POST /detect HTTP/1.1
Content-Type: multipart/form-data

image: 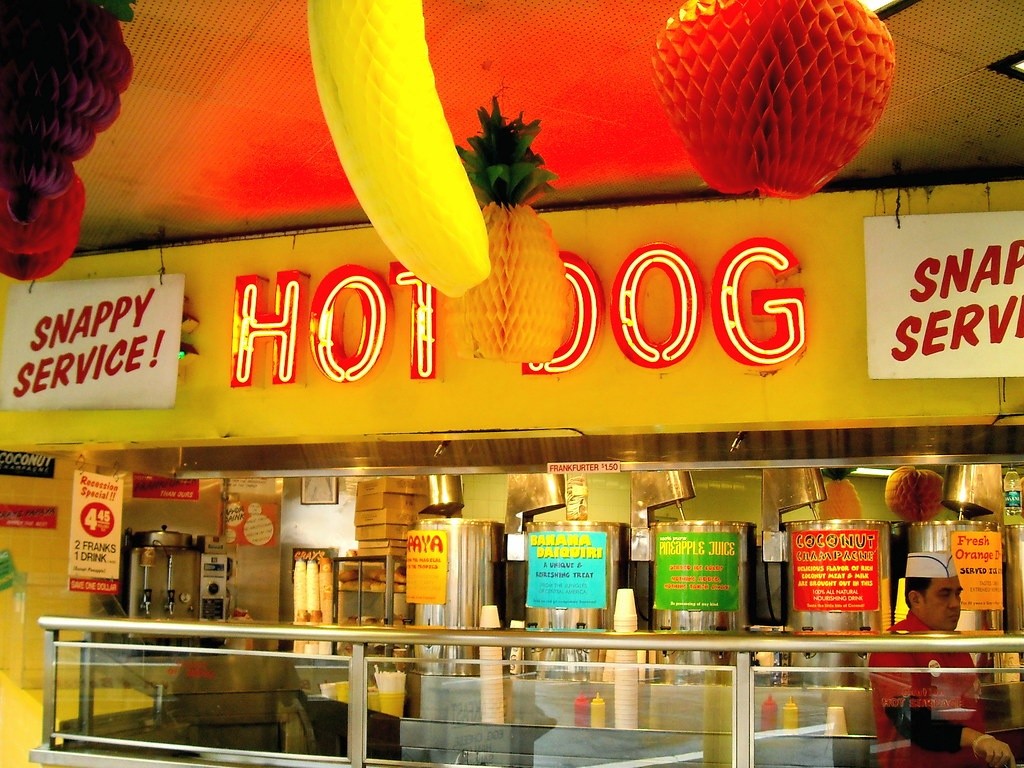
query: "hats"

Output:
[905,553,957,578]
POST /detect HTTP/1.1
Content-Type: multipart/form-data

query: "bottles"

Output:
[589,692,605,727]
[761,695,777,731]
[713,612,732,664]
[783,696,798,729]
[575,691,590,727]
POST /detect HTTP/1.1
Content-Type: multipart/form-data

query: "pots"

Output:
[124,525,194,548]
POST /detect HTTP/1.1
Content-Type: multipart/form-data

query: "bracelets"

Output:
[973,734,994,759]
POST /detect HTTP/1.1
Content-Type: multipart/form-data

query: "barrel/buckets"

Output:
[528,521,624,673]
[415,519,504,676]
[651,522,756,668]
[788,519,893,687]
[908,519,1004,667]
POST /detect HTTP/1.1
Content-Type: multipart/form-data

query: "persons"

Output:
[869,552,1016,768]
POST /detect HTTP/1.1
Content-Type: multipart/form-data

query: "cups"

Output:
[613,589,638,633]
[337,681,349,703]
[380,693,403,717]
[615,650,638,729]
[1003,464,1024,526]
[293,559,333,625]
[368,693,380,711]
[479,606,505,723]
[824,707,848,735]
[393,649,407,672]
[320,684,336,700]
